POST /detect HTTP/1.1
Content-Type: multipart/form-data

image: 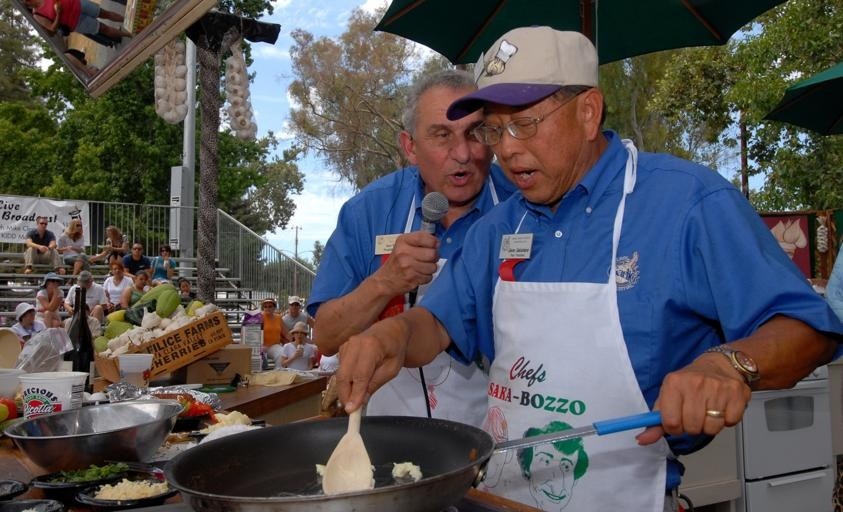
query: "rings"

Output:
[708,411,725,419]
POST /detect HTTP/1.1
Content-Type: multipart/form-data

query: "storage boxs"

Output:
[171,344,253,386]
[94,310,233,384]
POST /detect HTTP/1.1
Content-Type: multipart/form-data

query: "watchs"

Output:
[704,343,761,386]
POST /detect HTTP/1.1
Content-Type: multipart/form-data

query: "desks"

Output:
[202,364,329,427]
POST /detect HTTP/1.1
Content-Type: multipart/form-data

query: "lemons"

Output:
[0,404,9,421]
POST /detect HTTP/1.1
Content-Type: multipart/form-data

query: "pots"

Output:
[161,409,661,511]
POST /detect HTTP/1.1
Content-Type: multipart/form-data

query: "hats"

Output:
[446,25,599,120]
[16,303,34,321]
[289,296,301,305]
[40,272,65,288]
[288,322,309,335]
[264,299,276,305]
[77,270,92,287]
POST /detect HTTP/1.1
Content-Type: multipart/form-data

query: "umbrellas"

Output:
[762,61,842,138]
[373,0,787,67]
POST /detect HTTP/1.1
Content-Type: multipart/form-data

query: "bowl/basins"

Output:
[2,398,184,469]
[18,371,91,419]
[0,367,23,405]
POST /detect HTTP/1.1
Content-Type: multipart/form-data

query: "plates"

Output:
[1,470,183,512]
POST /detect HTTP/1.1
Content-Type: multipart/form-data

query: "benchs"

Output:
[1,252,265,341]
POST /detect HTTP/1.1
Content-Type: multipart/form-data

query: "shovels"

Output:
[322,398,373,496]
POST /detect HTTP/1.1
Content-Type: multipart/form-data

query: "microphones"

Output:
[407,192,449,306]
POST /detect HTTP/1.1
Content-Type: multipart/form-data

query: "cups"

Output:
[242,381,249,391]
[297,344,304,351]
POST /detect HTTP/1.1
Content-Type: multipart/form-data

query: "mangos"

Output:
[93,283,202,354]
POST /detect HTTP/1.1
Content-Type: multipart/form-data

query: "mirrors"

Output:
[11,0,218,98]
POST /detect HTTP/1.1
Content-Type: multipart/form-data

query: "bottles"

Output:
[117,352,154,393]
[61,286,95,395]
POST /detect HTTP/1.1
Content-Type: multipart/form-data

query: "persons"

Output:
[12,216,196,341]
[20,1,132,81]
[260,296,319,370]
[336,25,842,512]
[306,69,520,430]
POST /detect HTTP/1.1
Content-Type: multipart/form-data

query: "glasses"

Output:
[40,222,47,225]
[162,250,169,252]
[264,306,272,308]
[77,224,81,227]
[469,89,587,146]
